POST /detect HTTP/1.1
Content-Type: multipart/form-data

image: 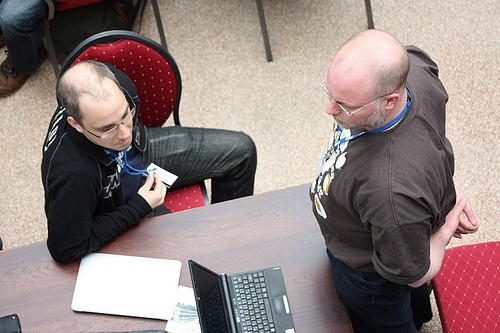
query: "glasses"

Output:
[74,87,137,140]
[319,79,391,117]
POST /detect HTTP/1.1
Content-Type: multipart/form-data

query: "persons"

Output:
[41,60,257,263]
[308,30,478,333]
[0,0,49,97]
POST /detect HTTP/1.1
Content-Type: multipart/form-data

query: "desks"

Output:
[0,183,355,333]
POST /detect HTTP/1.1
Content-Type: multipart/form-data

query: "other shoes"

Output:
[0,58,25,97]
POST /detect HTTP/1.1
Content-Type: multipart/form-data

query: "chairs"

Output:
[55,30,210,212]
[430,240,500,333]
[43,0,169,84]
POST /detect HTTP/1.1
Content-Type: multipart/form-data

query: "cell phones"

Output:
[0,313,22,333]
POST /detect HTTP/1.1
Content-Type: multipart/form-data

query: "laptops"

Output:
[187,259,297,333]
[71,251,182,320]
